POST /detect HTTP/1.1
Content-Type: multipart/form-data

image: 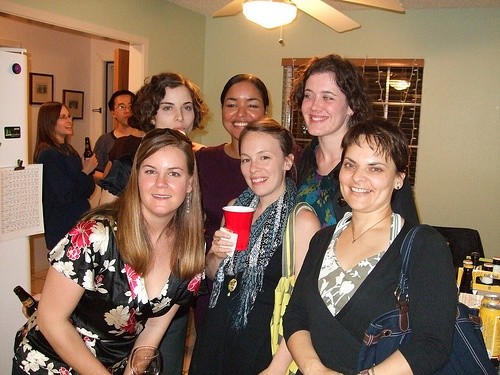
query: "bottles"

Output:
[13,286,42,317]
[84,137,96,175]
[460,251,500,294]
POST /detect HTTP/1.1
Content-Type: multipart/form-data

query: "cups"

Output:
[223,206,255,251]
[130,345,162,375]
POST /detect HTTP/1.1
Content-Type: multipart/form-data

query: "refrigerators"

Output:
[0,47,32,375]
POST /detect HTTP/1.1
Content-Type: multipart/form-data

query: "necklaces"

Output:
[350,214,393,243]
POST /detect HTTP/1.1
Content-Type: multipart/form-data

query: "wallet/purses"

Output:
[26,324,65,362]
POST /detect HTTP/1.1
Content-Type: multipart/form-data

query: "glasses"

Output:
[114,102,132,109]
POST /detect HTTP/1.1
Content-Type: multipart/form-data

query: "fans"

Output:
[211,0,405,33]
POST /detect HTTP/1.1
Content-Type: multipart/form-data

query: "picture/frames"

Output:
[63,89,84,120]
[29,72,54,104]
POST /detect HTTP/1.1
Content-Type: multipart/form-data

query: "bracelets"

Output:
[360,365,375,375]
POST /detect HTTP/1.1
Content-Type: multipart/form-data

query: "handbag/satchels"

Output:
[355,223,497,375]
[269,201,318,375]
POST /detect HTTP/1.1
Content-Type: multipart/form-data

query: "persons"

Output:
[282,117,458,375]
[11,73,321,375]
[289,54,421,225]
[33,102,98,251]
[92,90,135,184]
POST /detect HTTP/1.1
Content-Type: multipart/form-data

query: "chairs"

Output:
[433,226,486,268]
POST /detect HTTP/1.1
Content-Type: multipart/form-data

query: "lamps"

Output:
[243,0,297,44]
